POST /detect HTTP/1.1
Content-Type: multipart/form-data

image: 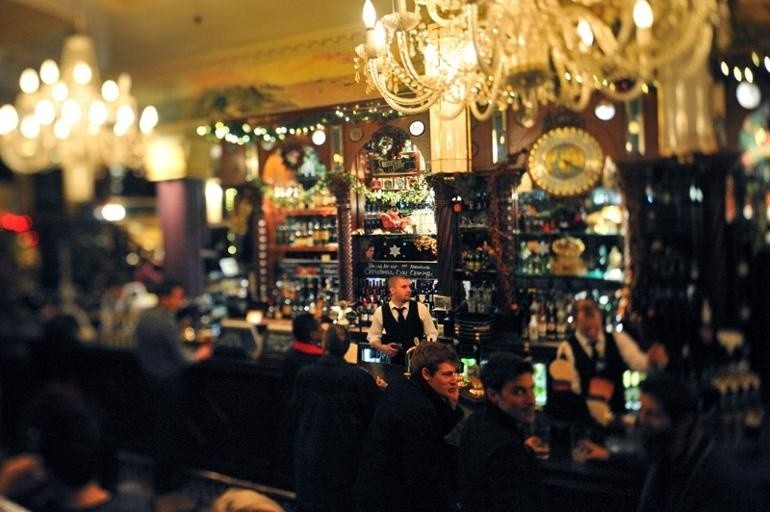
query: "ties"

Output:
[588,340,599,371]
[394,307,405,323]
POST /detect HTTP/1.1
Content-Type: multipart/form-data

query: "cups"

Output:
[529,423,552,460]
[570,426,592,465]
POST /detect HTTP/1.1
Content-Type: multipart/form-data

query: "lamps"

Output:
[353,0,720,129]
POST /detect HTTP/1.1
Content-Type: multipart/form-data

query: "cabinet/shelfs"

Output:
[252,150,766,360]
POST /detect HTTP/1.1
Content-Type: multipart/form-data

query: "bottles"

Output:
[523,288,572,341]
[460,248,495,270]
[359,279,389,326]
[275,270,338,320]
[520,241,549,275]
[274,217,337,246]
[467,286,493,313]
[365,204,387,232]
[409,280,437,312]
[596,245,622,270]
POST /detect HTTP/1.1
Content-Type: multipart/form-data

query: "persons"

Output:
[1,258,770,512]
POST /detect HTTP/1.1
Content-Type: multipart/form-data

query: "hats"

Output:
[114,281,158,311]
